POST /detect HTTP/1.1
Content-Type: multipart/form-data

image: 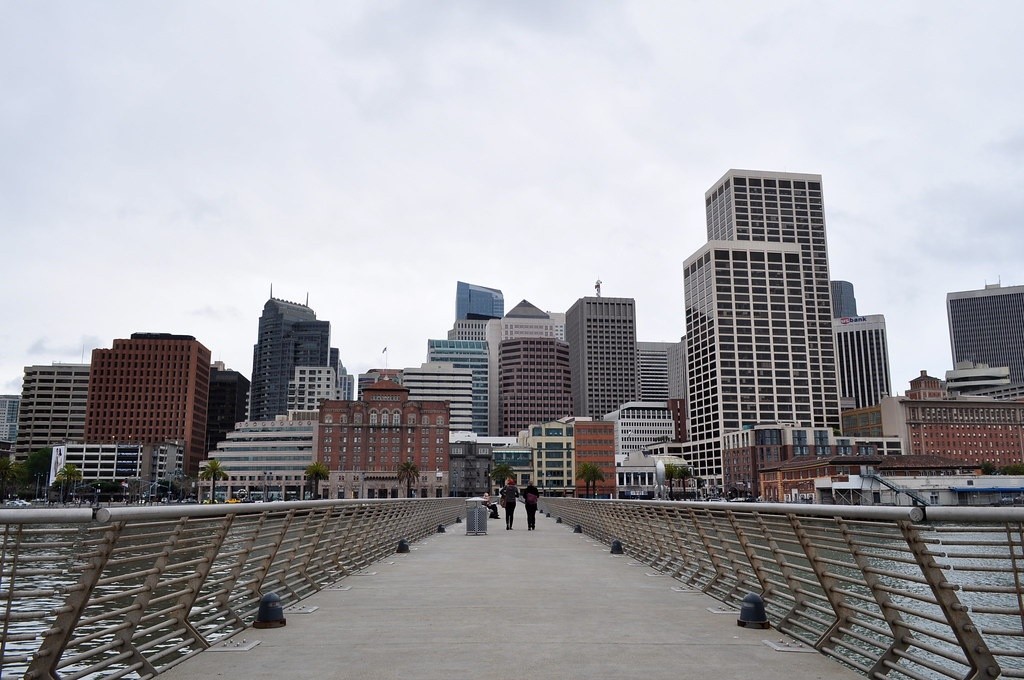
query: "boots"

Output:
[494,506,499,519]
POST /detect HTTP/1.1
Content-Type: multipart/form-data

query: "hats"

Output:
[507,476,515,485]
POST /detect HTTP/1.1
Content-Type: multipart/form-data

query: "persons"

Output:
[481,493,501,519]
[499,477,519,531]
[522,480,540,531]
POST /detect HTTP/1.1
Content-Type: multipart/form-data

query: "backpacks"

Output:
[526,486,538,505]
[506,486,515,507]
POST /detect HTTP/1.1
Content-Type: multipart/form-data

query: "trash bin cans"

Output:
[465,497,489,533]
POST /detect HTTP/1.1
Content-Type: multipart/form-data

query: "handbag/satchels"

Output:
[490,512,498,518]
[500,494,506,507]
